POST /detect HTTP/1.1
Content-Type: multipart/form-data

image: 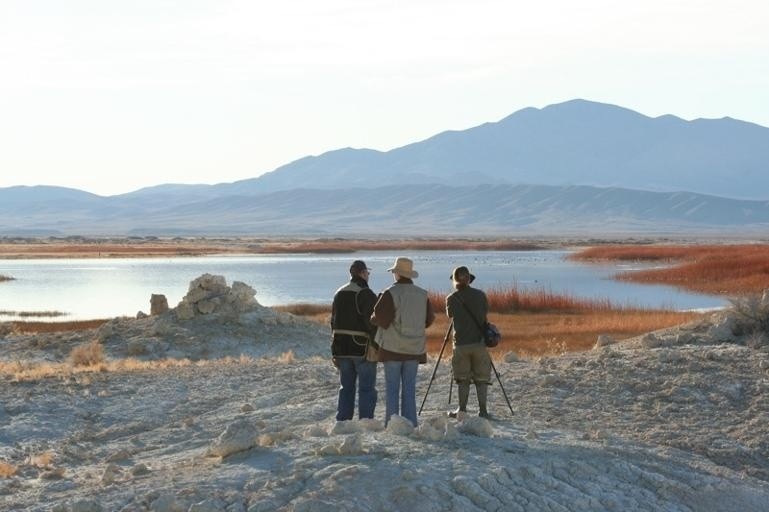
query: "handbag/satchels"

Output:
[482,319,500,348]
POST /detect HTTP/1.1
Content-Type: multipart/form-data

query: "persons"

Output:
[369,256,435,430]
[329,259,380,421]
[445,265,501,420]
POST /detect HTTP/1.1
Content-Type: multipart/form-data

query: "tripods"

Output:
[418,319,516,416]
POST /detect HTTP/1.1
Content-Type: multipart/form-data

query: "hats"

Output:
[385,255,419,281]
[350,259,373,276]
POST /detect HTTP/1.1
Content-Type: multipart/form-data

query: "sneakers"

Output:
[446,403,490,418]
